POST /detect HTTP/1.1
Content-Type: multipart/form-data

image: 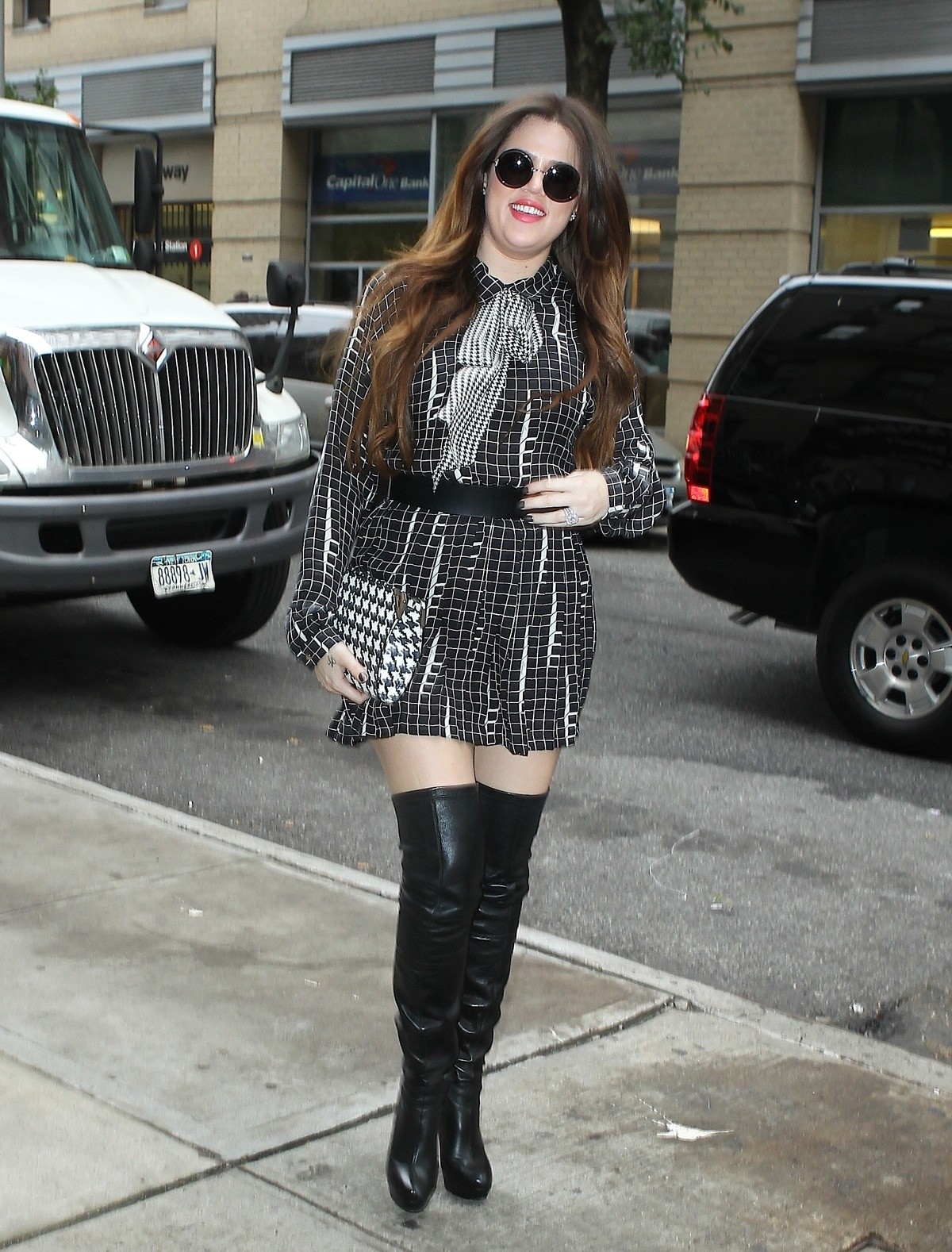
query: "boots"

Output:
[385,782,483,1213]
[434,781,550,1200]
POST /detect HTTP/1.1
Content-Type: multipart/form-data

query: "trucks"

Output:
[0,99,320,649]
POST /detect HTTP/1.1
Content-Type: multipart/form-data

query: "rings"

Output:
[564,508,579,526]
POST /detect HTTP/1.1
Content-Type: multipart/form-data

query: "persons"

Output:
[283,90,668,1217]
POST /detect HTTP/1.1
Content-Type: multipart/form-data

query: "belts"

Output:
[377,467,526,524]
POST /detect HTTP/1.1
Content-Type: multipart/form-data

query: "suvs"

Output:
[667,254,952,754]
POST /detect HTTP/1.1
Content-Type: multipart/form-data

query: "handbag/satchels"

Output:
[333,567,427,703]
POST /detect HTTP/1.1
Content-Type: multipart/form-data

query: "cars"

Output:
[220,302,355,450]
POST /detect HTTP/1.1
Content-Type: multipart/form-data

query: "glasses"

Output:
[491,149,582,203]
